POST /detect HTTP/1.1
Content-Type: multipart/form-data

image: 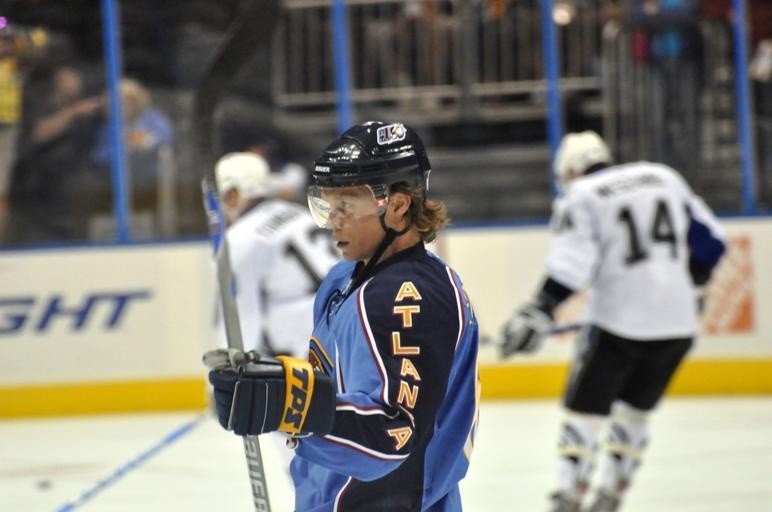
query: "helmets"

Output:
[201,153,270,200]
[553,129,612,194]
[307,119,432,229]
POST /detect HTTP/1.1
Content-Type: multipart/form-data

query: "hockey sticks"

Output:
[189,12,269,511]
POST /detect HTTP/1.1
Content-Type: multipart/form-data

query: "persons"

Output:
[213,151,342,359]
[2,1,772,244]
[202,119,478,512]
[498,129,728,512]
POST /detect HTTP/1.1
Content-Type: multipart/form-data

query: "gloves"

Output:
[498,298,556,359]
[201,347,336,436]
